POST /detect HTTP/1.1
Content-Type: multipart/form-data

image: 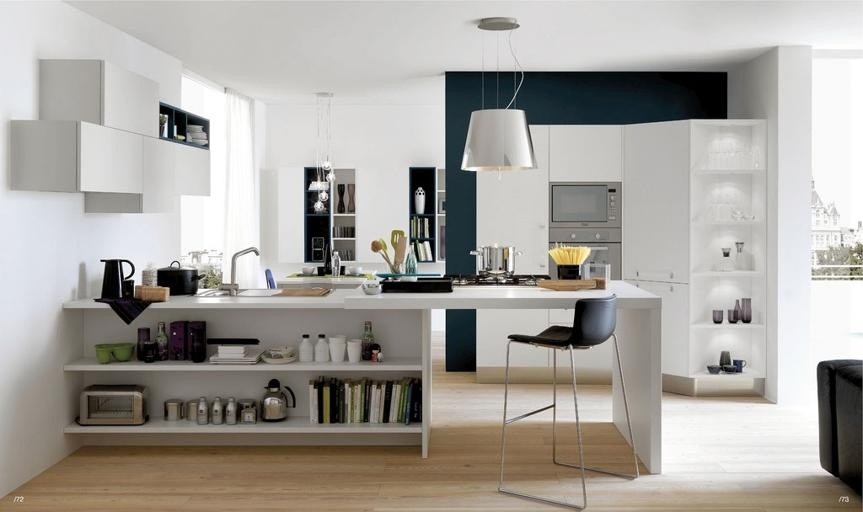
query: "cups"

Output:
[142,271,157,286]
[713,310,723,324]
[121,279,133,301]
[330,336,346,362]
[317,267,326,276]
[733,360,746,372]
[728,310,738,323]
[347,339,362,362]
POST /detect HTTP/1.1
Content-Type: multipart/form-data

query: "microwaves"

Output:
[549,182,622,228]
[78,384,150,425]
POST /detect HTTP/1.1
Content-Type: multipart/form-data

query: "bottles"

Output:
[225,397,236,424]
[144,341,155,362]
[717,248,735,271]
[155,321,169,361]
[298,334,313,361]
[315,334,330,361]
[163,399,184,420]
[734,299,742,319]
[720,351,731,365]
[211,396,223,424]
[239,399,257,424]
[187,399,199,420]
[372,350,379,361]
[198,396,209,425]
[405,242,418,274]
[362,321,375,360]
[734,242,746,270]
[742,298,752,323]
[331,252,341,278]
[137,327,150,359]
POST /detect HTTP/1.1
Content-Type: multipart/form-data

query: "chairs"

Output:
[264,268,279,289]
[496,294,640,511]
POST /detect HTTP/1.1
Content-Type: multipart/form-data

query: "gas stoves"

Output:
[444,274,551,287]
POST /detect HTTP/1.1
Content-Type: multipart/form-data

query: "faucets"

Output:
[219,247,260,296]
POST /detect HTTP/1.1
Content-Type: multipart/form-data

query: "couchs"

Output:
[817,360,863,501]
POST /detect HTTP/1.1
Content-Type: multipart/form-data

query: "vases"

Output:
[413,186,426,215]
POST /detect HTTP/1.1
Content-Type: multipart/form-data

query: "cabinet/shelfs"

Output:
[409,166,446,263]
[10,59,211,214]
[62,279,662,475]
[304,166,355,263]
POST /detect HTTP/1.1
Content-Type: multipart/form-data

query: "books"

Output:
[307,372,422,426]
[415,241,433,260]
[332,225,355,238]
[410,215,429,240]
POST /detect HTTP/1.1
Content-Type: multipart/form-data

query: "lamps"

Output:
[309,90,337,213]
[460,16,537,182]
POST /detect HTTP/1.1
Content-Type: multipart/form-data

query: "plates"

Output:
[261,354,296,364]
[187,125,208,146]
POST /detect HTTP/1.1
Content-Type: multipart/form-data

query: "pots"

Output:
[157,261,205,295]
[469,246,522,275]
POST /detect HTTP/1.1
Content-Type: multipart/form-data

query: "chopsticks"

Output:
[548,244,591,264]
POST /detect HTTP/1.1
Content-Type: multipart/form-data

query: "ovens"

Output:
[549,242,622,280]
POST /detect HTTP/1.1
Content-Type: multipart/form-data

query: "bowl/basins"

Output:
[301,266,315,275]
[362,284,382,296]
[723,365,737,375]
[266,345,294,358]
[707,366,722,374]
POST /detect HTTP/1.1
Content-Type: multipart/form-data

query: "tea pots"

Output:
[260,378,297,422]
[100,258,135,302]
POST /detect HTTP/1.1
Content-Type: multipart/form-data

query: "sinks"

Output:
[198,288,281,296]
